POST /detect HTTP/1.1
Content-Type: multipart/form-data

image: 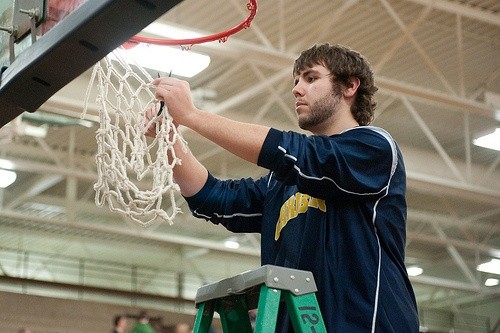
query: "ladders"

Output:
[191,263,329,333]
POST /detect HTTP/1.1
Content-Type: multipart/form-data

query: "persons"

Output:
[143,43,420,333]
[109,309,191,332]
